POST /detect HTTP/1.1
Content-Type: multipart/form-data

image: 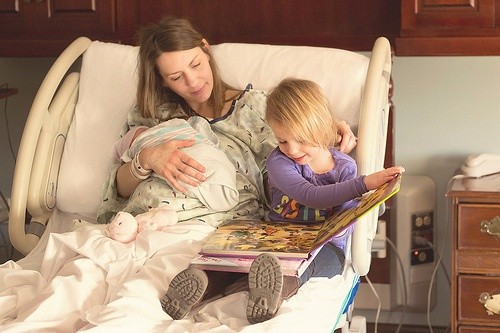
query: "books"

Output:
[189,170,403,277]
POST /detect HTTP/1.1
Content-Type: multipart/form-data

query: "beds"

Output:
[0,36,393,333]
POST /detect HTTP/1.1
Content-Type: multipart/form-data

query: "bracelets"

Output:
[130,150,153,180]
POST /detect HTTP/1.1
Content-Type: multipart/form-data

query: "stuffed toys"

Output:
[105,204,179,243]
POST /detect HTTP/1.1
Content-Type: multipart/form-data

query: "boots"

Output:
[161,268,226,320]
[246,253,299,324]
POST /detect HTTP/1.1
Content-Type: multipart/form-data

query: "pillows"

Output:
[55,40,370,217]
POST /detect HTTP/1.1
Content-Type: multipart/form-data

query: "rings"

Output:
[355,137,358,142]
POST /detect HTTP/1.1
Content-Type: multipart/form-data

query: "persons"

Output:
[161,79,405,325]
[117,116,239,212]
[96,16,359,229]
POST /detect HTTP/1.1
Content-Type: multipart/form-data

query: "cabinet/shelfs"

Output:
[445,191,500,333]
[0,0,500,58]
